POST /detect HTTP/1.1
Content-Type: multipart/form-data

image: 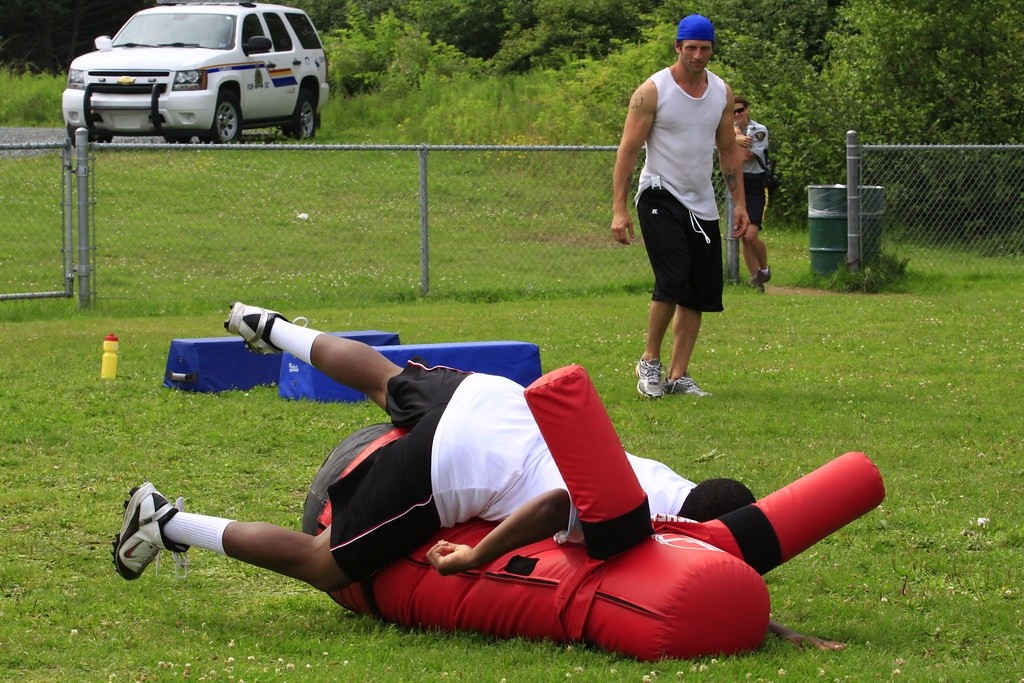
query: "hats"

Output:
[676,15,715,42]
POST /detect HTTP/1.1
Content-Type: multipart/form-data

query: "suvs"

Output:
[61,0,330,143]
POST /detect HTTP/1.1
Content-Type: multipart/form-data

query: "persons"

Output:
[609,14,751,401]
[109,300,758,594]
[732,95,773,294]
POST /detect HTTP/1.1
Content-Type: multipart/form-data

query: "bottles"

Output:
[102,333,118,380]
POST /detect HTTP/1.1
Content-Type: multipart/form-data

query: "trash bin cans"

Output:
[807,183,886,276]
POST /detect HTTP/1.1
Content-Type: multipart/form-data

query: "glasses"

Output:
[734,106,747,115]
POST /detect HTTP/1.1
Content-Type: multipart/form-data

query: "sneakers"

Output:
[109,479,189,580]
[633,355,665,400]
[222,300,290,357]
[661,375,712,397]
[750,266,772,292]
